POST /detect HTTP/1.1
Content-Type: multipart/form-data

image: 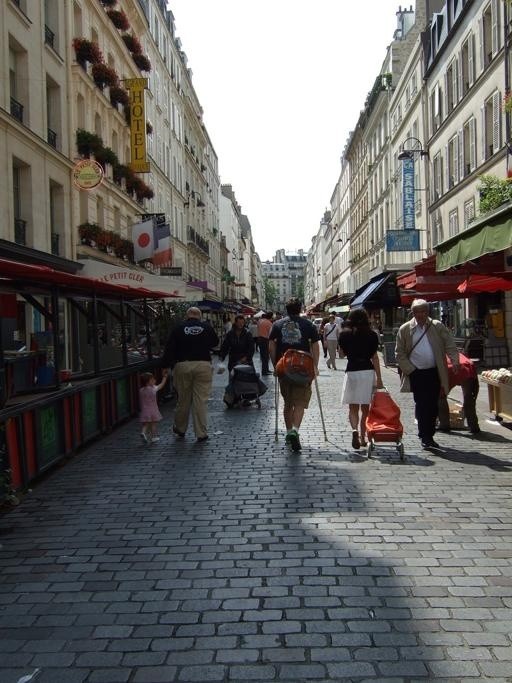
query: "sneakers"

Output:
[352,430,361,449]
[151,437,159,442]
[141,433,148,443]
[197,435,208,442]
[286,430,302,451]
[421,441,439,450]
[173,424,185,437]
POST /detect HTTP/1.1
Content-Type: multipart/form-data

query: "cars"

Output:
[313,317,323,332]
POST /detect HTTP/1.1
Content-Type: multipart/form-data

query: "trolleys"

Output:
[366,386,404,460]
[224,356,267,410]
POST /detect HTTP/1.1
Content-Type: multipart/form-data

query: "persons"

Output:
[134,292,482,459]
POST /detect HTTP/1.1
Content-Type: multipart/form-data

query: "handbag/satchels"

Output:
[275,349,315,388]
